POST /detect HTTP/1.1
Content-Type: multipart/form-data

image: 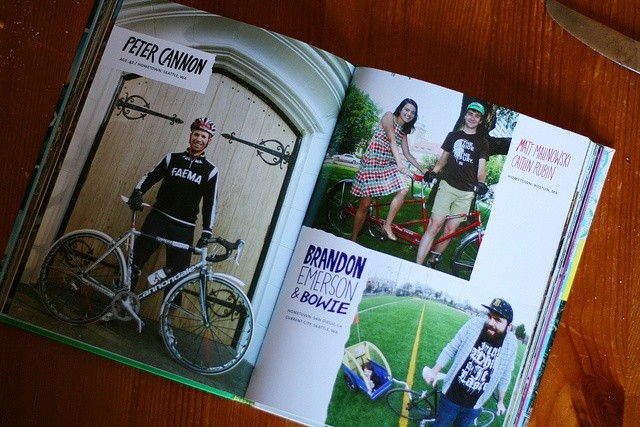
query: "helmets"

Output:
[466,102,485,119]
[190,117,216,138]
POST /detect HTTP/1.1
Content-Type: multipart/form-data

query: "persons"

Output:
[97,115,221,346]
[350,98,427,243]
[414,100,491,265]
[423,296,517,426]
[362,364,382,391]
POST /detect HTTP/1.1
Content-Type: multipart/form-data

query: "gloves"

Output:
[424,171,437,183]
[476,182,487,195]
[193,237,208,255]
[127,193,144,212]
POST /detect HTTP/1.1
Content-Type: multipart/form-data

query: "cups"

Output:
[148,267,175,286]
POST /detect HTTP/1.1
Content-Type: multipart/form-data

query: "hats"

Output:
[482,298,513,322]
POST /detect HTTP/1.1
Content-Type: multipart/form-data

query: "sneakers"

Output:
[158,325,178,347]
[98,311,120,322]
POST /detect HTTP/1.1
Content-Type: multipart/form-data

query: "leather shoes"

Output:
[381,225,397,241]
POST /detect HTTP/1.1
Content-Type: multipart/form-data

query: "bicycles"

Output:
[40,194,254,375]
[326,172,489,276]
[386,366,498,426]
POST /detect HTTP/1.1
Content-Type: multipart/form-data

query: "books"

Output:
[2,0,615,426]
